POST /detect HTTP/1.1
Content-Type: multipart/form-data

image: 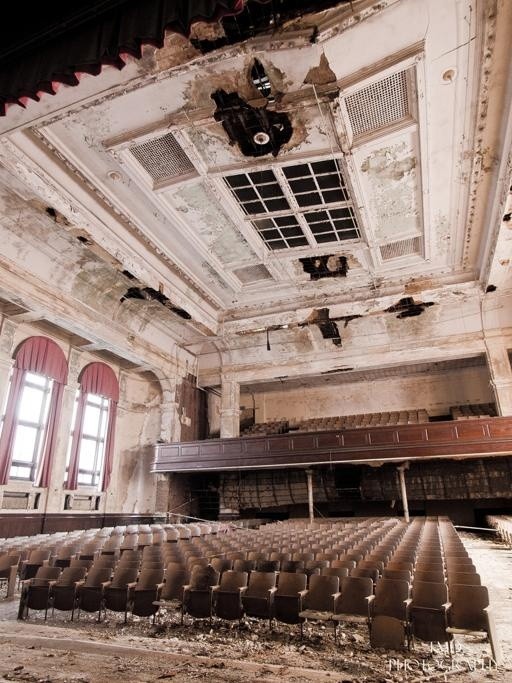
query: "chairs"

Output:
[289,409,429,433]
[240,421,287,436]
[450,403,500,419]
[487,514,512,549]
